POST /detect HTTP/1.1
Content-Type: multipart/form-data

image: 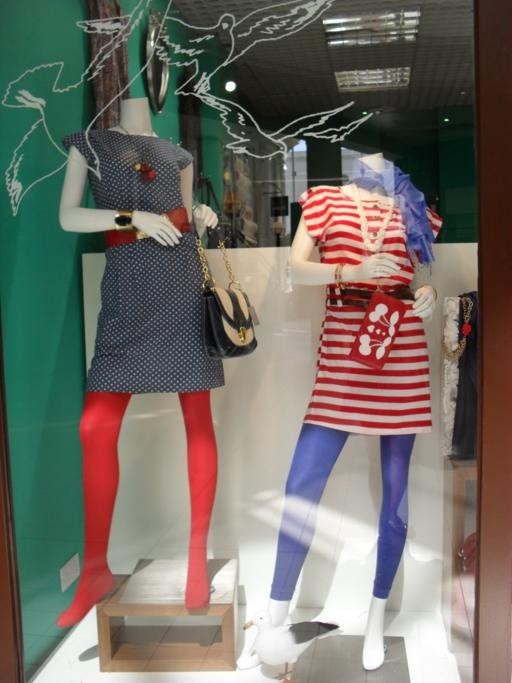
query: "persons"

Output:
[234,153,444,672]
[56,97,219,627]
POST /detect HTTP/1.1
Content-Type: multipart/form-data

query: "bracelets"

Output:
[114,210,133,230]
[334,263,347,290]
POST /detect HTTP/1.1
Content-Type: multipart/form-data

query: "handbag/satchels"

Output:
[349,290,405,371]
[193,278,258,358]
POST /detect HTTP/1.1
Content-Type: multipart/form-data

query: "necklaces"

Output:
[118,124,157,180]
[351,183,396,253]
[441,296,473,361]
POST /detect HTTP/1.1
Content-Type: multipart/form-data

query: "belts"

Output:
[106,206,191,246]
